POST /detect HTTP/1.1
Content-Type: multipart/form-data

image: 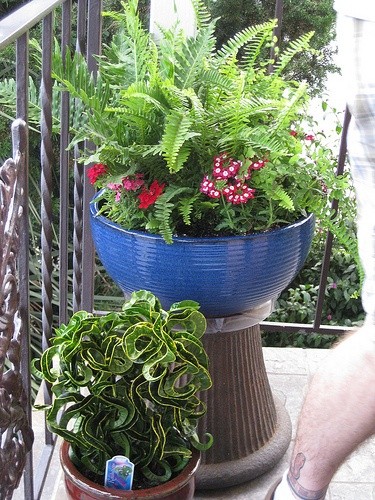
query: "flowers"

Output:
[51,0,364,281]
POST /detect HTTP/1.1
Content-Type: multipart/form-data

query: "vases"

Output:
[90,194,313,315]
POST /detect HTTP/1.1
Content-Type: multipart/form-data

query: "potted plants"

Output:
[31,289,213,500]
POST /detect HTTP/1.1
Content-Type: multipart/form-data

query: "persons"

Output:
[263,0,375,500]
